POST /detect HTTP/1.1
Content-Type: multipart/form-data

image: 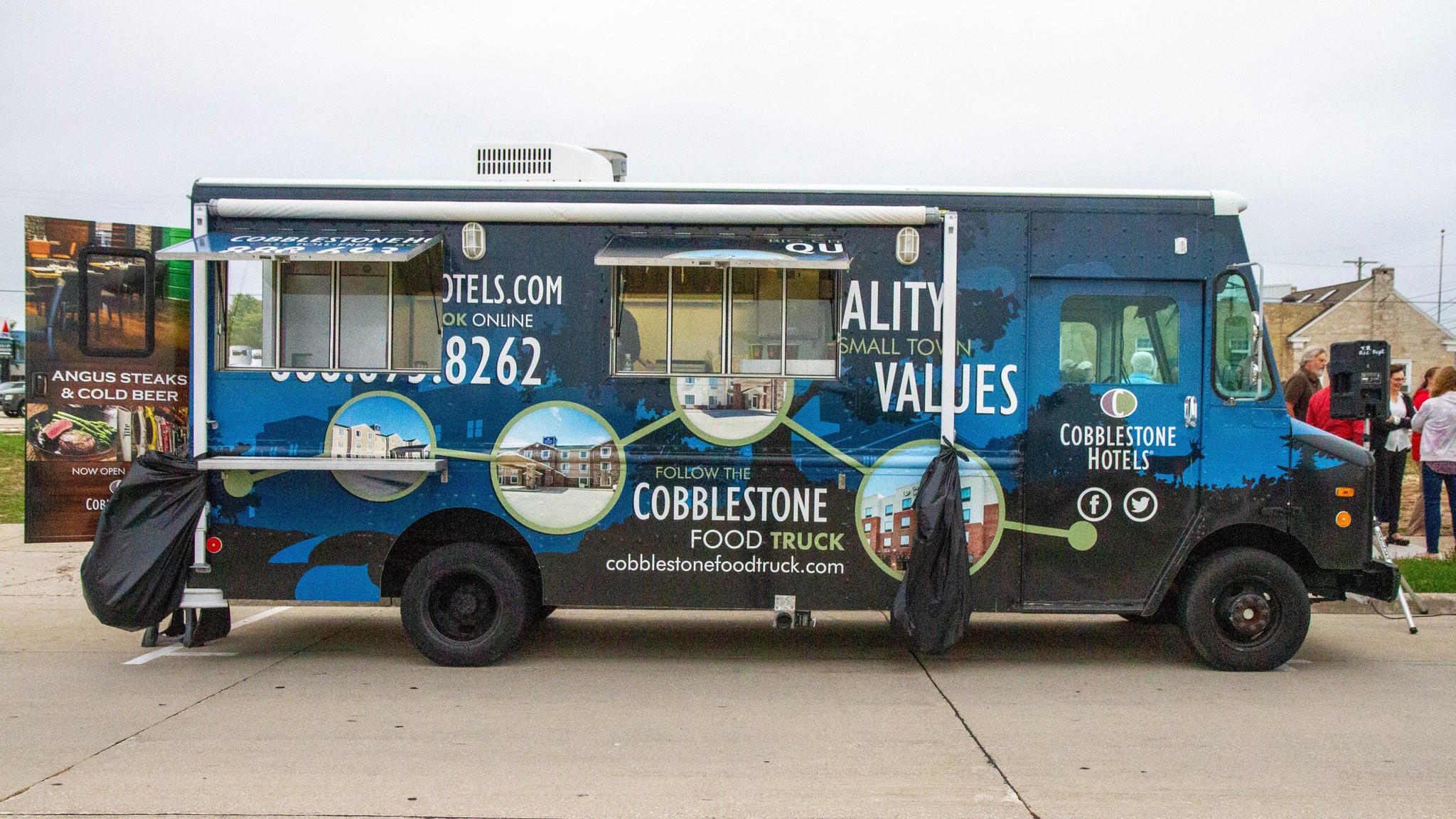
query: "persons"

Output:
[1406,367,1444,537]
[618,299,640,371]
[1062,359,1096,384]
[1369,364,1417,546]
[1305,359,1365,447]
[1283,344,1327,424]
[1410,366,1456,560]
[1120,351,1162,385]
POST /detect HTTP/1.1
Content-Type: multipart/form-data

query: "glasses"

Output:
[1392,377,1407,382]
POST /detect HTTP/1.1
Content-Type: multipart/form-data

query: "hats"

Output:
[1061,359,1077,370]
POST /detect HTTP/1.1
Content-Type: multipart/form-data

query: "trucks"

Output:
[175,143,1404,668]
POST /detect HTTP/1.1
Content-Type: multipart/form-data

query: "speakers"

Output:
[1328,339,1391,421]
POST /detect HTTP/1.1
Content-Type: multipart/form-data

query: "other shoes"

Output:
[1385,535,1410,546]
[1413,552,1437,561]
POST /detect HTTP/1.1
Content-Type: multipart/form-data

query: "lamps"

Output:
[896,226,920,267]
[461,221,486,261]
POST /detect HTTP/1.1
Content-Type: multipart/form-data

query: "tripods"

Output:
[1370,420,1428,634]
